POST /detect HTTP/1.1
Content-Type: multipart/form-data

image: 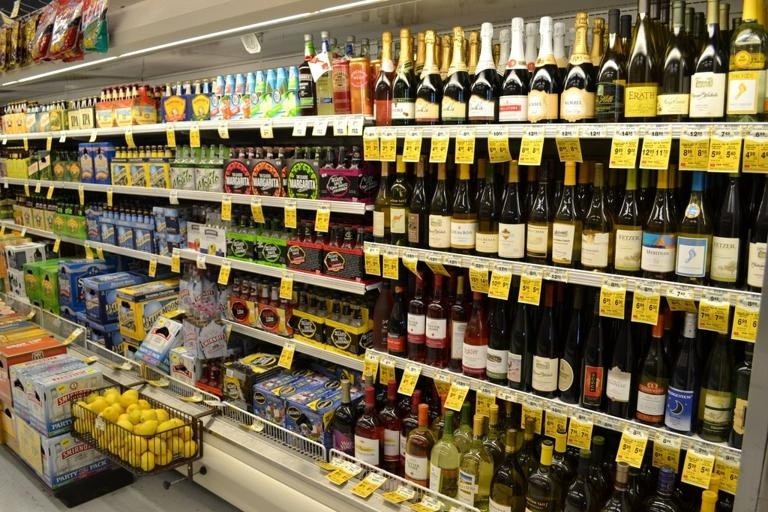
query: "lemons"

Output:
[73,388,198,472]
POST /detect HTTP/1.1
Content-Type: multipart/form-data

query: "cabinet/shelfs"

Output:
[0,3,767,510]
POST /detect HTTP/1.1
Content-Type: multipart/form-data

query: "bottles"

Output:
[2,0,767,512]
